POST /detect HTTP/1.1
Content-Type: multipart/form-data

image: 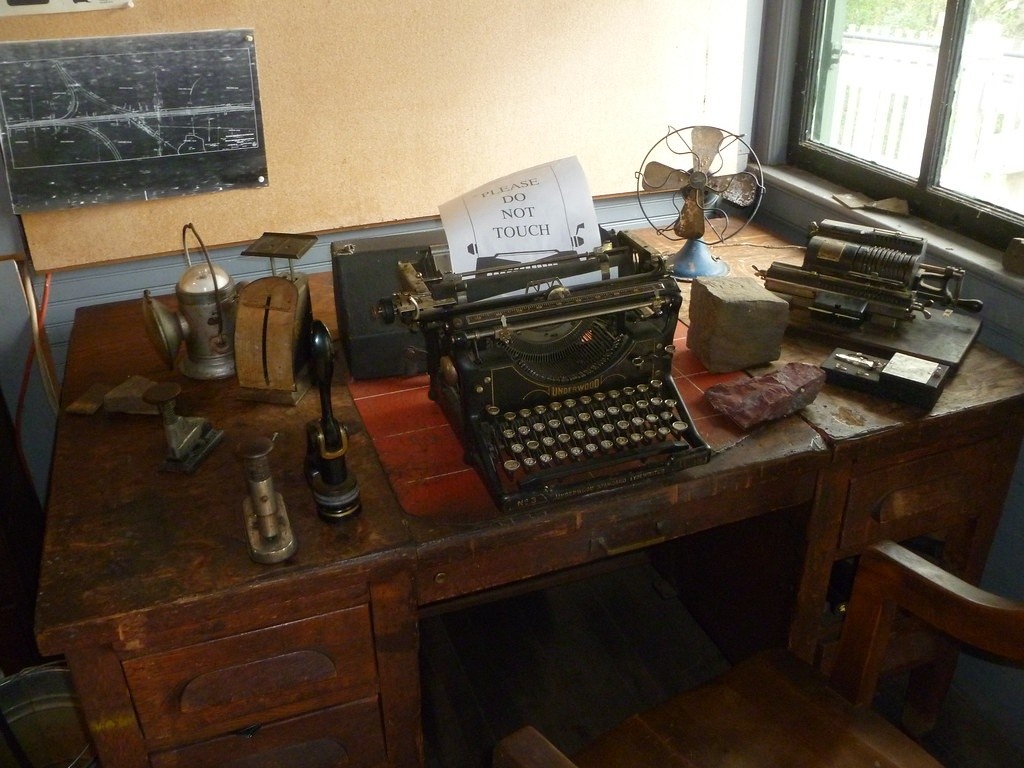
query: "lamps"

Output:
[141,222,236,381]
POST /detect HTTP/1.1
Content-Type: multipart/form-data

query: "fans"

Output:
[635,124,767,283]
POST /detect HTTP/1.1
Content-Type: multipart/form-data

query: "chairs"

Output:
[488,543,1024,768]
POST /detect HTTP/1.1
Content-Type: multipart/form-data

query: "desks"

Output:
[33,218,1024,767]
